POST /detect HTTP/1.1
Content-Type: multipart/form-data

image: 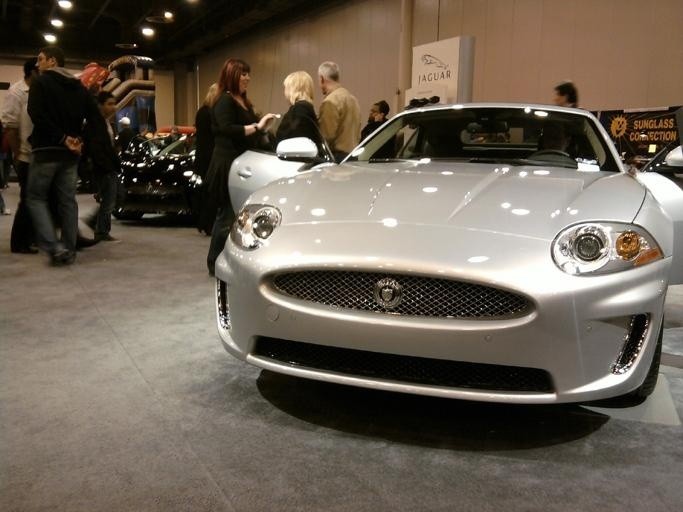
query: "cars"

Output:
[212,100,683,407]
[639,137,683,190]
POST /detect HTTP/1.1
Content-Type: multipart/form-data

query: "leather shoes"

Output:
[17,247,38,253]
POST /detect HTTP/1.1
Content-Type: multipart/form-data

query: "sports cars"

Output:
[101,133,202,231]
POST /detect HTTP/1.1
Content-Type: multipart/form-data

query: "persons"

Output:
[193,82,219,236]
[275,71,320,154]
[0,59,40,254]
[316,61,362,164]
[553,83,577,109]
[205,58,282,276]
[26,47,88,266]
[359,101,395,159]
[0,128,12,216]
[397,123,430,158]
[83,91,120,242]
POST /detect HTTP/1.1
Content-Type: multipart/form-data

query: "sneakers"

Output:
[98,234,121,244]
